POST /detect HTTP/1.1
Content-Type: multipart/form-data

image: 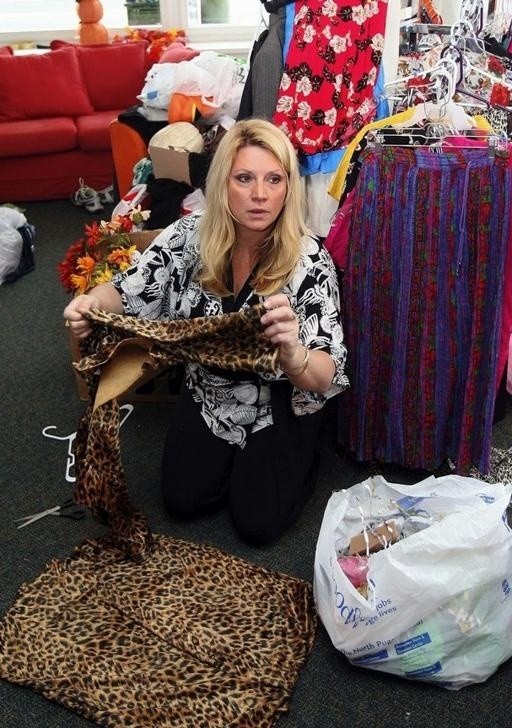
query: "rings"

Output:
[65,319,69,329]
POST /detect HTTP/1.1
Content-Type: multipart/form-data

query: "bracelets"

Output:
[280,345,311,380]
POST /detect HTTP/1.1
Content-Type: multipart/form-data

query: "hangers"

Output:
[40,403,134,485]
[381,16,511,132]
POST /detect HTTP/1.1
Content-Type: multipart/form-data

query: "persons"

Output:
[64,117,350,545]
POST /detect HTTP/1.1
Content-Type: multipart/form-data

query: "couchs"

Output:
[0,41,201,203]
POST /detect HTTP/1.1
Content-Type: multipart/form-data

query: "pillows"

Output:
[0,41,199,120]
